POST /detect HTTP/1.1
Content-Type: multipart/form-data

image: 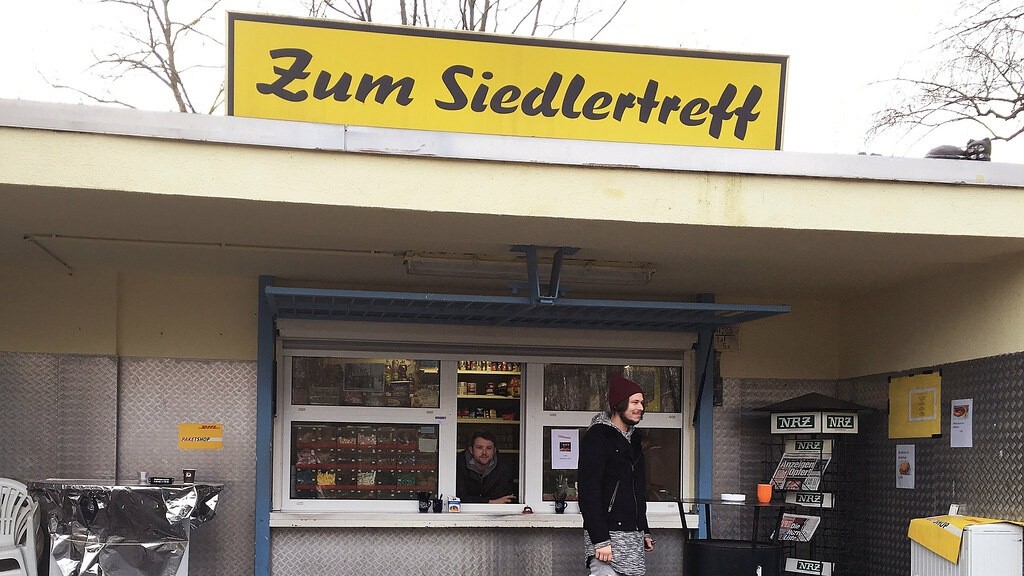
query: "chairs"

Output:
[0,476,41,576]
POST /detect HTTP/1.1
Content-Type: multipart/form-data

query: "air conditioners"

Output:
[910,520,1023,576]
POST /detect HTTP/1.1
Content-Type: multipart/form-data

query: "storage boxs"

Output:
[292,362,438,500]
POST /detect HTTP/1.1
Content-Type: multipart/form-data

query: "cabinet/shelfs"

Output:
[418,370,521,453]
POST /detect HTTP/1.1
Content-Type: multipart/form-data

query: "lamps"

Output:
[399,250,657,286]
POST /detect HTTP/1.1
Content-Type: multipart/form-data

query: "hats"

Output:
[609,375,643,407]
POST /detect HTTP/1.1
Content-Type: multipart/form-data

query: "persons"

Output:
[456,429,519,505]
[576,373,657,576]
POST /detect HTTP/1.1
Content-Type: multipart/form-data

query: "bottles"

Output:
[400,360,407,378]
[385,361,392,384]
[393,360,399,380]
[407,374,414,394]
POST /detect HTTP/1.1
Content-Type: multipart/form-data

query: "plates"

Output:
[952,406,966,418]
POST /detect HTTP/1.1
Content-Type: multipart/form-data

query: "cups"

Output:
[419,499,431,513]
[555,500,567,513]
[137,471,150,484]
[183,469,196,484]
[433,500,443,512]
[757,484,772,502]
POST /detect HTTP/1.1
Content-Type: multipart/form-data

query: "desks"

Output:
[26,479,225,576]
[669,498,801,576]
